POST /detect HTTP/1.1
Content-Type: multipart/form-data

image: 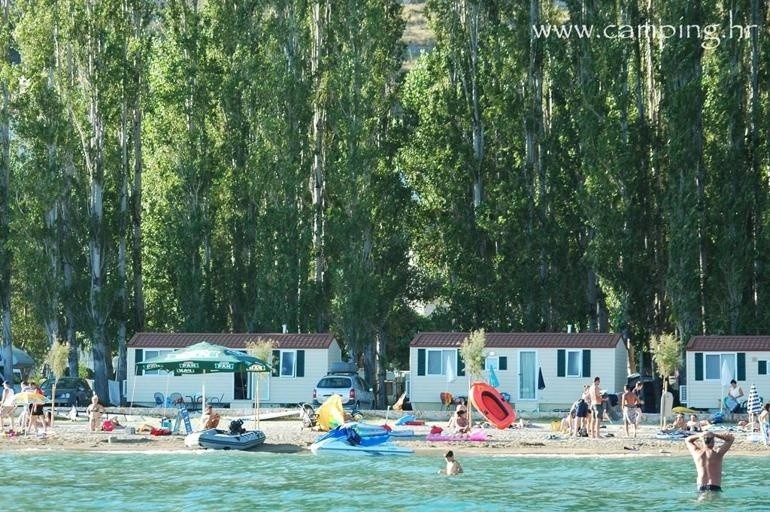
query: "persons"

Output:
[661,379,770,446]
[558,376,644,439]
[685,430,735,503]
[438,450,463,477]
[447,397,471,435]
[86,395,105,431]
[200,405,220,430]
[0,380,53,436]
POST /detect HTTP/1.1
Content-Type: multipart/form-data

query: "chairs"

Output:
[440,393,455,411]
[154,392,224,414]
[742,397,763,413]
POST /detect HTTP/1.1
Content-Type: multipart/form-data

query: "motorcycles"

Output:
[307,414,416,458]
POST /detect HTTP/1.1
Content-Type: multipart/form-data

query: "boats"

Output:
[183,428,266,451]
[470,381,517,430]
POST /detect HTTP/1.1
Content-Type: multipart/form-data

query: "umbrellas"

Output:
[488,364,500,389]
[136,341,272,427]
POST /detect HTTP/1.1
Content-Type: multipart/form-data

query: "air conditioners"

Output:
[679,385,686,404]
[122,380,127,397]
[405,380,410,397]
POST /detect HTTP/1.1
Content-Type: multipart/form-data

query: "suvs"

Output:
[312,362,374,410]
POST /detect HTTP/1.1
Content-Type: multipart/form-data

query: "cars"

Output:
[0,368,22,401]
[28,377,93,415]
[627,372,680,413]
